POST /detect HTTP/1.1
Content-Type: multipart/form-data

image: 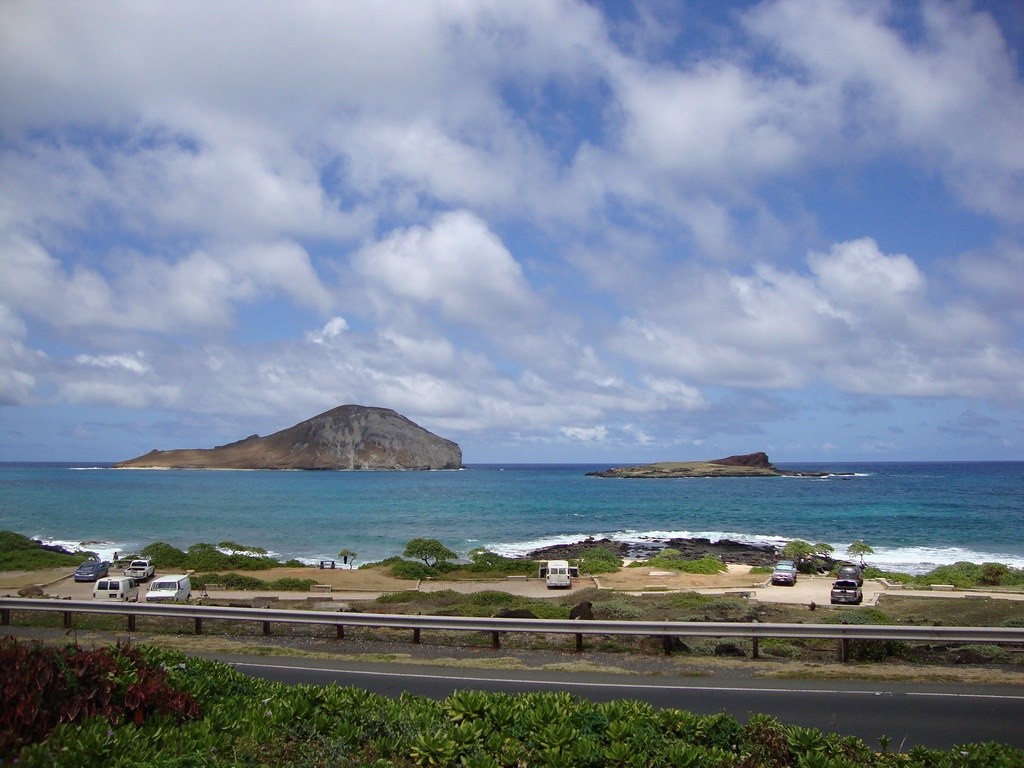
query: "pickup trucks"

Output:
[123,559,157,582]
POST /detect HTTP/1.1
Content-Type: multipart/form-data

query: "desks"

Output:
[320,561,336,565]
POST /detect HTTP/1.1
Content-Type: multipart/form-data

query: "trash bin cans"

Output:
[569,567,578,577]
[539,568,547,578]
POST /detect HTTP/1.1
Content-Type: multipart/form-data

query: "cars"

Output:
[74,561,109,582]
[830,580,864,604]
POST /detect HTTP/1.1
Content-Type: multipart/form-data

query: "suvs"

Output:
[144,574,191,604]
[771,560,801,586]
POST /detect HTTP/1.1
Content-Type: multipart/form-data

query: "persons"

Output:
[112,551,119,561]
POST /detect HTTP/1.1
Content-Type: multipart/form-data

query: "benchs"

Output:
[310,585,332,592]
[318,564,335,569]
[205,583,227,590]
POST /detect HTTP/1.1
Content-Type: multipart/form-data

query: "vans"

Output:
[92,576,140,602]
[545,560,572,588]
[837,562,865,587]
